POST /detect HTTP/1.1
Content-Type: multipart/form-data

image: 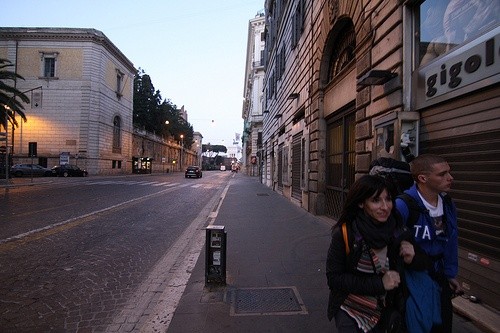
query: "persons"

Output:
[394,153,461,333]
[326,174,428,333]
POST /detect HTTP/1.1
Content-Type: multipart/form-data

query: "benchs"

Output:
[451,293,500,333]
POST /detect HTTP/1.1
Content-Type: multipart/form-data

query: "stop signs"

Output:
[0,146,7,152]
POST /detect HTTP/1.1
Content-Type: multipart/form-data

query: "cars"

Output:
[58,164,88,177]
[185,166,203,178]
[10,163,51,178]
[49,165,61,176]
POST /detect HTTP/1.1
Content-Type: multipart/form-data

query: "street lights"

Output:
[179,134,184,172]
[161,119,170,172]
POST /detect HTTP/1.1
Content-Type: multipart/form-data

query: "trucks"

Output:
[220,166,226,171]
